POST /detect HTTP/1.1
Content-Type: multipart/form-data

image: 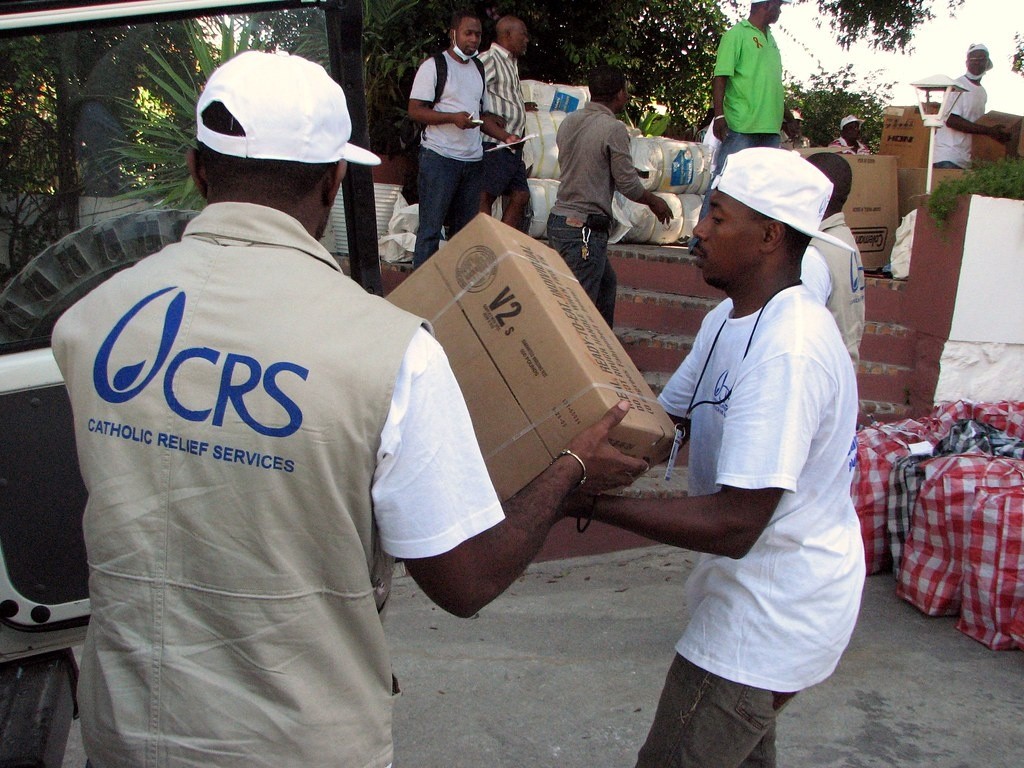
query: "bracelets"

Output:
[576,490,596,534]
[714,114,725,120]
[558,448,586,485]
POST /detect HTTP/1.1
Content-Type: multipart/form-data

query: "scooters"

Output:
[0,0,398,768]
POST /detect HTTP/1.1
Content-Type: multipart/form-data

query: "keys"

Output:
[581,247,589,260]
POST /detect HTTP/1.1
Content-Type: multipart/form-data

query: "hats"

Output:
[196,49,383,168]
[967,43,993,71]
[750,0,792,4]
[841,114,866,130]
[784,109,804,121]
[716,145,858,258]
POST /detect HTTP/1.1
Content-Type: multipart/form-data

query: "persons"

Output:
[932,43,1012,170]
[828,114,870,155]
[406,11,525,272]
[779,109,810,150]
[556,145,867,768]
[800,151,865,378]
[688,0,802,256]
[546,63,675,331]
[694,99,870,156]
[50,50,652,768]
[67,24,154,231]
[474,14,540,230]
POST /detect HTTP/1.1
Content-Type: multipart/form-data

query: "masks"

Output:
[451,29,480,62]
[965,67,987,81]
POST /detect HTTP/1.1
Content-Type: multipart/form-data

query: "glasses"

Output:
[970,57,985,62]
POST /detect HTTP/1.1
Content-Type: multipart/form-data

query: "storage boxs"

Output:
[790,102,1024,274]
[377,212,675,517]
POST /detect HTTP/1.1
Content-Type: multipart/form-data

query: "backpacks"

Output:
[369,52,485,158]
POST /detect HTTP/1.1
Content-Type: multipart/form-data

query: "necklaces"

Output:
[665,280,804,479]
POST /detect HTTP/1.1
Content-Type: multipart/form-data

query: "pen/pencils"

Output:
[468,111,476,119]
